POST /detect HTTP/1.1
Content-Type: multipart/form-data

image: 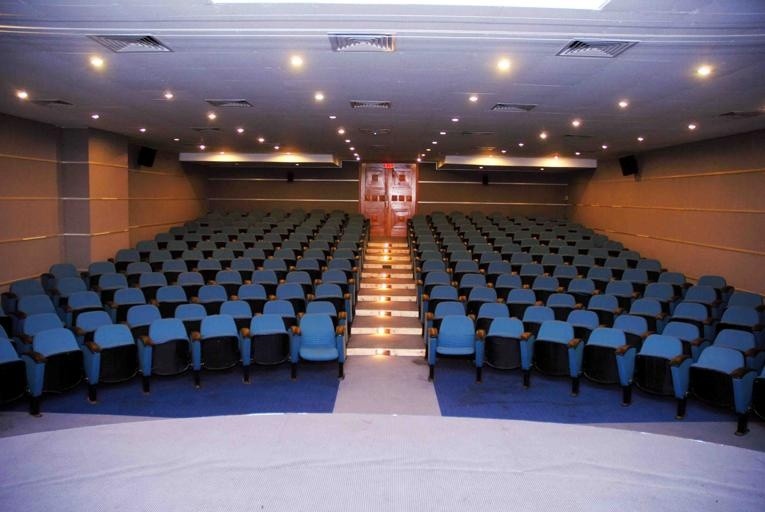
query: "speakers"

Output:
[618,155,639,176]
[482,173,488,186]
[288,171,292,181]
[137,149,155,168]
